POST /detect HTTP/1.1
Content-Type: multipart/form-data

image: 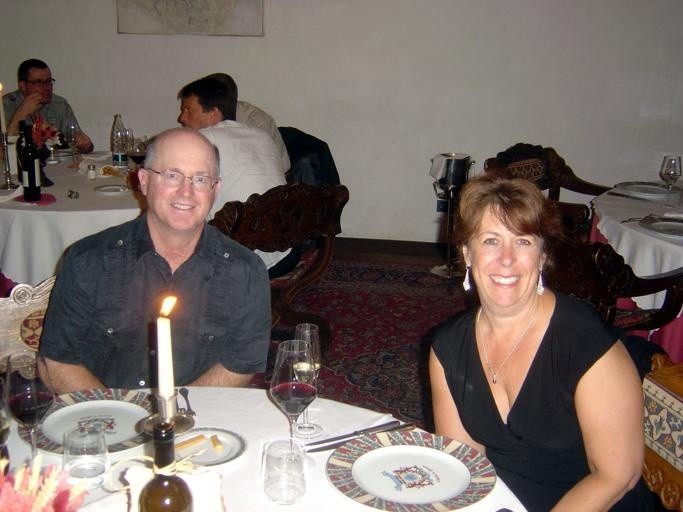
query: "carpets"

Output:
[248,260,478,436]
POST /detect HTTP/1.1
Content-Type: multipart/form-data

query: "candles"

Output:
[155,317,174,421]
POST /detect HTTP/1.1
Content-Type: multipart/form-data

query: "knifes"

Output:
[305,420,401,447]
[606,190,654,205]
[304,419,417,454]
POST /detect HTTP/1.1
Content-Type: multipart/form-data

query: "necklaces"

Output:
[478,299,541,384]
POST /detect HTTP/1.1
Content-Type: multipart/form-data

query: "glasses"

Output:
[147,166,218,193]
[24,78,54,86]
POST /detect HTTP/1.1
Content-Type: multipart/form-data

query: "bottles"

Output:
[86,163,96,181]
[136,422,194,512]
[110,111,126,170]
[12,120,42,203]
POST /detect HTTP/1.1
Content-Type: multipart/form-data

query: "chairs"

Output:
[0,274,57,405]
[543,219,683,330]
[624,337,683,512]
[279,126,343,236]
[482,142,613,230]
[209,184,349,356]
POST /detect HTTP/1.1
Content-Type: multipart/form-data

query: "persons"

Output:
[427,172,671,512]
[177,76,298,278]
[38,128,270,393]
[1,59,94,154]
[208,74,292,181]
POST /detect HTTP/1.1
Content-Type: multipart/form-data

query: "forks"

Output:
[173,387,196,417]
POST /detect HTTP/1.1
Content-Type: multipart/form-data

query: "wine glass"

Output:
[3,350,57,465]
[657,154,679,209]
[66,122,83,168]
[268,337,320,469]
[291,322,325,439]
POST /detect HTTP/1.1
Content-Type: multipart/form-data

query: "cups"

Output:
[0,377,9,449]
[59,424,110,494]
[126,127,150,169]
[262,441,304,505]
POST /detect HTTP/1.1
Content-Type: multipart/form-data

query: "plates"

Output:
[172,427,248,467]
[638,215,682,241]
[92,184,129,193]
[325,431,498,512]
[614,179,677,198]
[17,387,159,454]
[52,151,71,159]
